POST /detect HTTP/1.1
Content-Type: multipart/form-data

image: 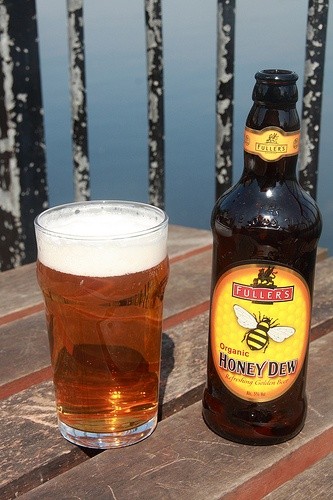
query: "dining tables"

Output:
[0,219,333,500]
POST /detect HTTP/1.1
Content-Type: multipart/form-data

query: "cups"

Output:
[34,200,176,452]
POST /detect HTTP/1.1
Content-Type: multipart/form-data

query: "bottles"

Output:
[199,67,323,445]
